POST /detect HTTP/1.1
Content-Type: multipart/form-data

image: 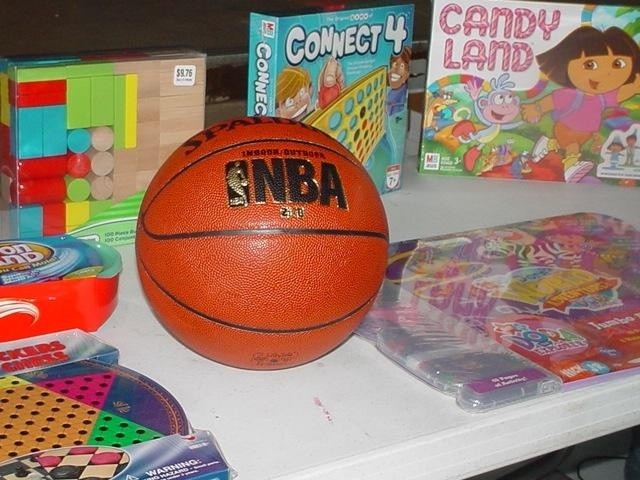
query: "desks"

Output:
[1,100,640,480]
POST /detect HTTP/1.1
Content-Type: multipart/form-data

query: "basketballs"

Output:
[135,117,389,371]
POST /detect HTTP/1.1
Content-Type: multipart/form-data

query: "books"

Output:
[245,1,415,198]
[417,1,639,189]
